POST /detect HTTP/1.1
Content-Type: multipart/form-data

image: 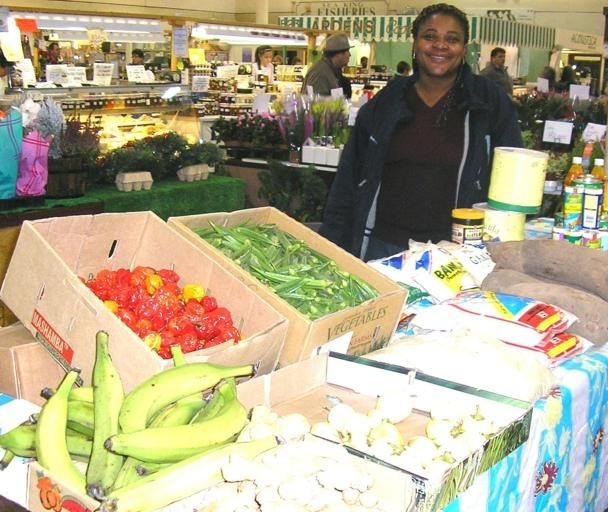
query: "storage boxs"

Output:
[0,207,288,400]
[227,345,539,511]
[22,412,430,510]
[166,202,414,370]
[1,323,62,401]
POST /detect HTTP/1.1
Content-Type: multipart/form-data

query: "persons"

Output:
[255,45,273,70]
[292,57,302,65]
[386,60,411,86]
[131,49,153,73]
[475,46,513,98]
[581,63,591,75]
[40,42,62,82]
[533,65,558,90]
[360,57,368,68]
[556,66,577,90]
[273,55,283,68]
[0,47,8,80]
[300,32,356,99]
[315,2,527,264]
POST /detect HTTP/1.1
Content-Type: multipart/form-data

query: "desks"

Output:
[0,209,608,512]
[0,171,249,329]
[223,160,341,222]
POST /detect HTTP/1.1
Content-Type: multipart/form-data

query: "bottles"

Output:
[565,157,584,187]
[590,158,606,181]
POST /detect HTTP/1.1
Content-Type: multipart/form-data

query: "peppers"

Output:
[76,267,246,359]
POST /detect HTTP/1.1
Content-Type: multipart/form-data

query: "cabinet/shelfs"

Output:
[199,114,236,147]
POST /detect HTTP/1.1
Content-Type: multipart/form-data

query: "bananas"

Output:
[1,330,279,512]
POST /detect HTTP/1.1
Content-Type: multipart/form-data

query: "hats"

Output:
[320,32,356,54]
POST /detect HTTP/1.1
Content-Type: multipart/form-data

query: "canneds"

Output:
[449,208,486,246]
[551,173,604,250]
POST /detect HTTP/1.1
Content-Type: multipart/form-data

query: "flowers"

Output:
[208,84,357,171]
[11,90,228,186]
[516,83,608,193]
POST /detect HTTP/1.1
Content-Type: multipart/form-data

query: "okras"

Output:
[192,218,381,320]
